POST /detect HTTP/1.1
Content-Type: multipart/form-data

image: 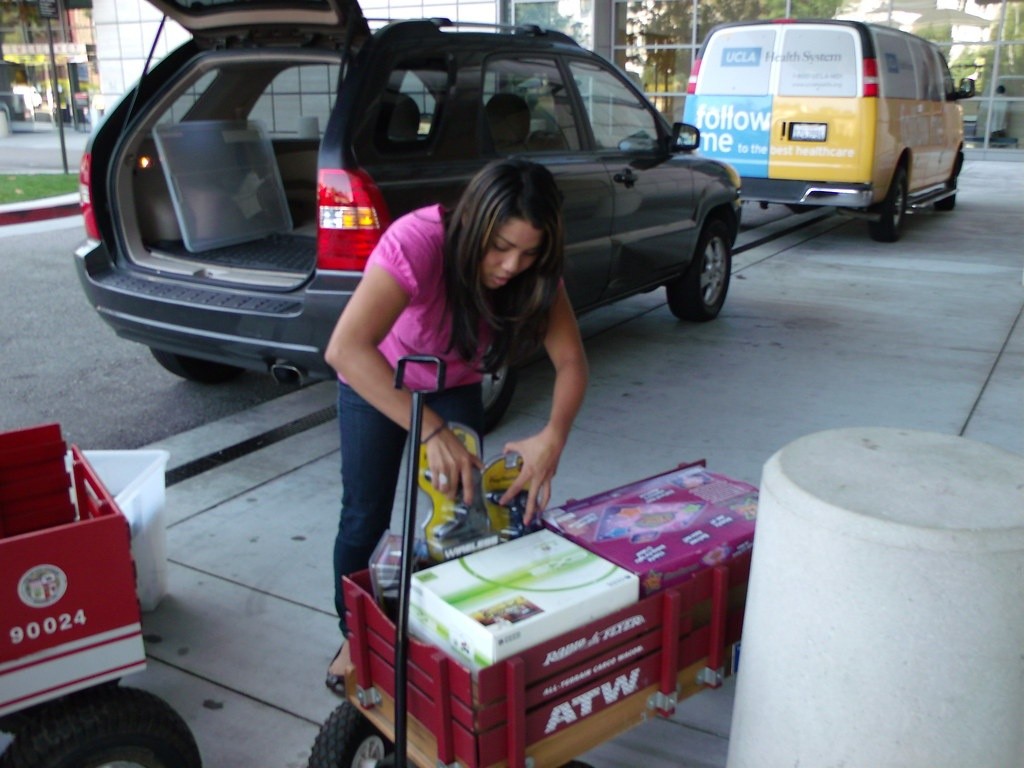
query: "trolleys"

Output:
[309,354,759,768]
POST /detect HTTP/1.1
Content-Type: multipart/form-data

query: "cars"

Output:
[0,58,95,127]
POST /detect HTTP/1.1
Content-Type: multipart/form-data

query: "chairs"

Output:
[556,99,600,151]
[387,94,421,141]
[484,93,532,155]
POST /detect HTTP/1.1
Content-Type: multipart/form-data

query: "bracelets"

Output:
[421,423,446,443]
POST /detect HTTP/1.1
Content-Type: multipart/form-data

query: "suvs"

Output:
[72,0,746,444]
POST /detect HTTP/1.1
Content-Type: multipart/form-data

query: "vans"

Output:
[683,16,978,245]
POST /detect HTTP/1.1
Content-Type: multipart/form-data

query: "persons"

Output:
[324,161,589,698]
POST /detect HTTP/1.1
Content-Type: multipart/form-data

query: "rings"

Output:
[439,474,449,484]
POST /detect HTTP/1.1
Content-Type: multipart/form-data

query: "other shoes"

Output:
[326,641,350,690]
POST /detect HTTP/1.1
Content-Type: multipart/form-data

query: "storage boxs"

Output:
[0,423,147,718]
[80,450,170,612]
[341,459,758,768]
